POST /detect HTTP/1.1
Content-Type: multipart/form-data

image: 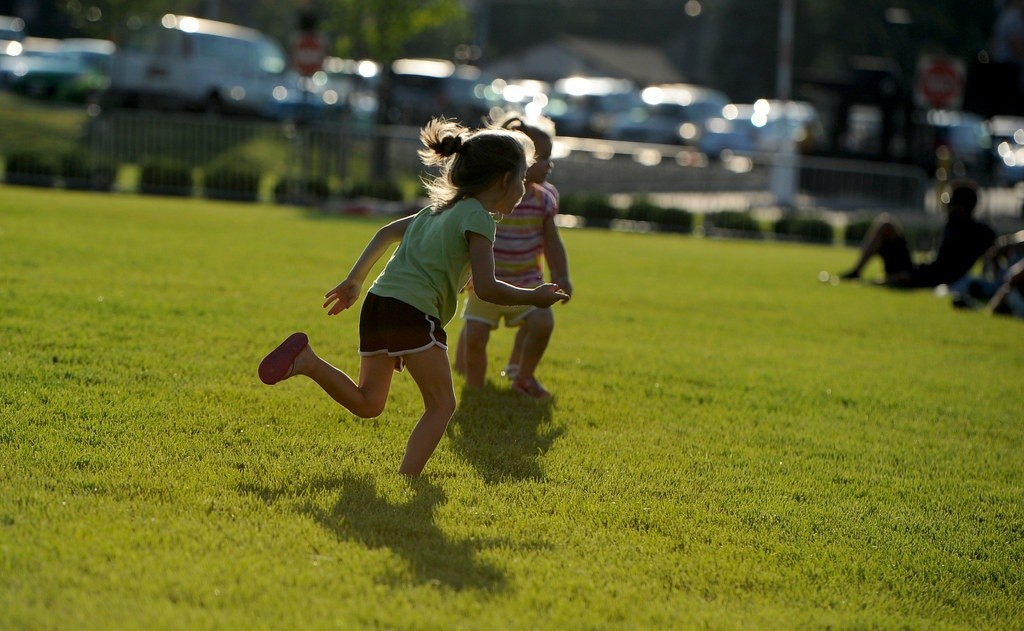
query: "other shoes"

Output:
[511,373,549,399]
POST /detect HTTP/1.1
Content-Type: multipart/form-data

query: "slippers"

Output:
[258,332,308,385]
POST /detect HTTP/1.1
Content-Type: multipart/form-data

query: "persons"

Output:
[839,178,998,290]
[258,113,570,477]
[949,195,1024,322]
[453,97,572,397]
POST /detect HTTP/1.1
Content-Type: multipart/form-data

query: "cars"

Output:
[1,5,1024,187]
